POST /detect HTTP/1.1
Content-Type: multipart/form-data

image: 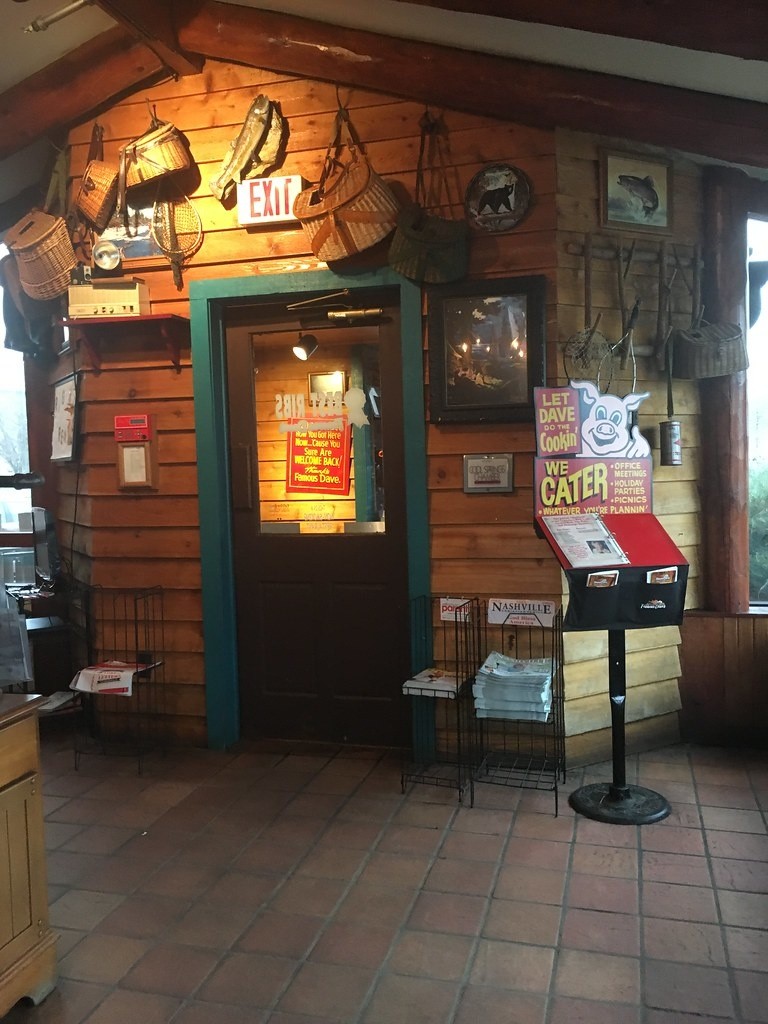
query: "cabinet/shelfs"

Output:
[392,594,570,818]
[5,584,165,771]
[0,691,64,1020]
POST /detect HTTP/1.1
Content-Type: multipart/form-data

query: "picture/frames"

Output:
[426,274,549,426]
[597,148,674,237]
[49,373,81,464]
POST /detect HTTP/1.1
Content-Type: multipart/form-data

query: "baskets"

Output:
[0,207,78,320]
[119,123,190,189]
[668,318,749,379]
[293,163,402,261]
[388,211,470,283]
[76,159,119,229]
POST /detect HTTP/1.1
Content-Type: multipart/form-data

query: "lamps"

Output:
[92,241,124,278]
